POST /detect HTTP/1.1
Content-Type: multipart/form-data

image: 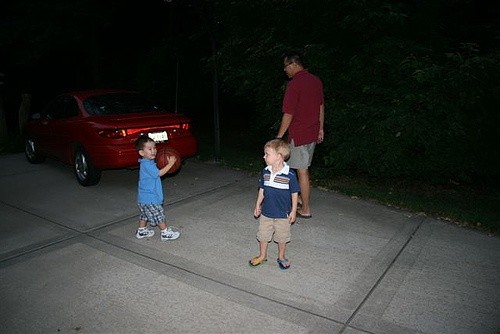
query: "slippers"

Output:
[277,258,290,269]
[296,209,312,219]
[249,257,267,267]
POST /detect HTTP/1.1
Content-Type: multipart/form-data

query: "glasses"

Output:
[284,62,293,68]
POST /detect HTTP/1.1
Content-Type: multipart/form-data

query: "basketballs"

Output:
[156,148,181,174]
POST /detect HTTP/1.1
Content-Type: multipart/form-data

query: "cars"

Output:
[24,83,200,187]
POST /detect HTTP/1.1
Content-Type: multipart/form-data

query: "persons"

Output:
[249,140,299,270]
[135,135,181,242]
[276,50,324,219]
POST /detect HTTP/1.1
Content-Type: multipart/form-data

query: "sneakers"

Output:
[136,228,154,239]
[160,229,180,240]
